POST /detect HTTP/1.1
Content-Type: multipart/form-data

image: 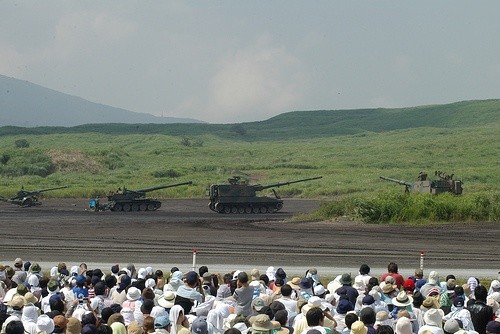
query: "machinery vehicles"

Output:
[206,175,322,216]
[107,180,193,211]
[378,169,464,198]
[7,185,69,207]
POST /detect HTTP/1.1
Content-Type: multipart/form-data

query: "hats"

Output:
[217,264,500,334]
[14,258,23,266]
[0,261,212,334]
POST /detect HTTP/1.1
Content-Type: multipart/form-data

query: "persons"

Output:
[95,196,99,212]
[0,258,500,334]
